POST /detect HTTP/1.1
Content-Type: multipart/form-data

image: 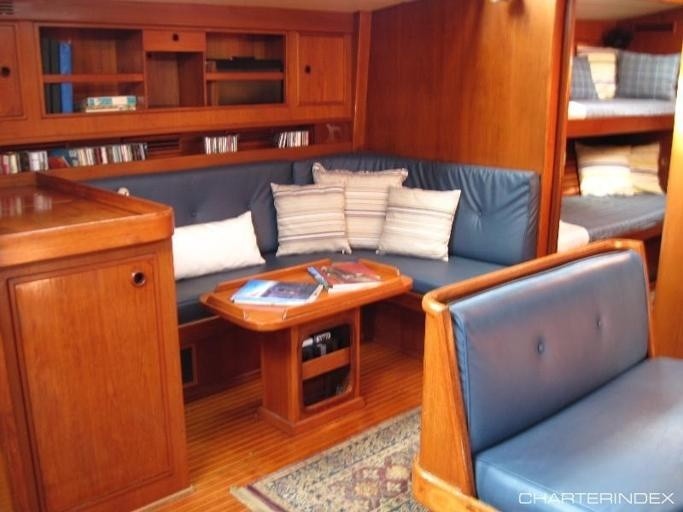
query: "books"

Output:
[230,279,325,306]
[1,33,311,177]
[314,262,382,287]
[306,266,380,293]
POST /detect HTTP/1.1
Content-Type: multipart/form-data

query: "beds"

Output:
[553,94,678,251]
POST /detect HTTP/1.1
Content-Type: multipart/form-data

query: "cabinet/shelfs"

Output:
[0,239,192,512]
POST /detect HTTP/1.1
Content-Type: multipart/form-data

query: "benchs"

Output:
[78,152,542,409]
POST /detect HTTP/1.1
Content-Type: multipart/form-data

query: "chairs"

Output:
[408,238,681,511]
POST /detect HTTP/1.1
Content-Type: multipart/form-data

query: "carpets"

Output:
[227,403,425,512]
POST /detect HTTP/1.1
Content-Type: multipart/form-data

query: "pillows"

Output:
[567,44,681,101]
[170,164,462,281]
